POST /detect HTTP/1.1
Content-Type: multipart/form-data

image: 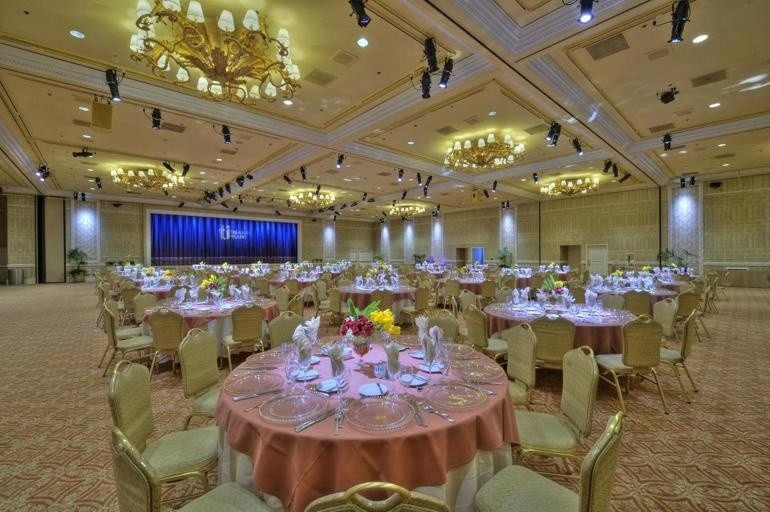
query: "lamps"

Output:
[105,69,120,101]
[204,191,211,198]
[362,192,367,201]
[618,172,631,183]
[210,192,217,201]
[328,206,335,211]
[218,187,223,198]
[163,160,175,173]
[577,0,594,24]
[389,206,426,218]
[182,162,190,176]
[603,159,612,173]
[232,207,237,213]
[152,108,161,130]
[421,71,431,99]
[572,139,584,156]
[690,176,695,185]
[72,147,93,157]
[533,172,538,183]
[379,218,384,223]
[318,208,325,213]
[129,0,302,106]
[484,189,489,198]
[539,176,600,197]
[283,174,292,184]
[177,202,185,208]
[402,190,407,200]
[73,191,78,200]
[335,211,340,215]
[40,171,50,180]
[112,201,122,207]
[398,169,404,182]
[368,198,375,202]
[423,37,439,73]
[316,185,320,196]
[37,165,46,174]
[236,176,244,186]
[340,203,347,209]
[114,157,186,195]
[225,184,232,193]
[432,211,440,218]
[417,172,421,184]
[288,191,336,209]
[438,57,453,88]
[336,154,345,169]
[545,121,561,147]
[663,134,671,151]
[425,176,432,188]
[492,180,497,193]
[221,202,228,209]
[443,131,527,172]
[246,174,253,180]
[680,178,686,188]
[350,201,359,207]
[275,210,281,216]
[81,192,86,201]
[506,200,509,208]
[95,177,102,190]
[423,187,427,196]
[382,211,387,216]
[238,194,243,204]
[204,196,211,204]
[667,0,692,42]
[348,0,371,28]
[333,215,336,221]
[612,163,619,177]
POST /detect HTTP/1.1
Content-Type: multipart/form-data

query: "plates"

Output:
[245,349,292,365]
[345,394,416,433]
[439,340,474,359]
[409,350,439,360]
[314,377,348,393]
[296,370,319,381]
[416,360,445,374]
[449,359,504,384]
[385,343,406,351]
[421,380,486,410]
[399,374,428,387]
[357,383,388,397]
[258,390,328,426]
[223,369,285,396]
[307,355,322,368]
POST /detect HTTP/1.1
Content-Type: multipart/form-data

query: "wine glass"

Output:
[399,364,414,401]
[333,366,351,423]
[437,347,452,390]
[284,355,302,397]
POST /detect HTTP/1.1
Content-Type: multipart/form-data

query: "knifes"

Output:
[238,366,278,370]
[292,405,342,433]
[229,387,284,401]
[376,384,383,394]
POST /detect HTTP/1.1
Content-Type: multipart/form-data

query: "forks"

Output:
[421,401,456,422]
[333,408,340,436]
[333,405,341,439]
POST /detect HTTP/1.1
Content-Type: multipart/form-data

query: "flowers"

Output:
[198,274,219,299]
[370,307,401,345]
[541,273,568,294]
[164,272,182,283]
[642,265,652,272]
[611,270,623,276]
[203,275,230,293]
[340,298,381,338]
[542,274,568,305]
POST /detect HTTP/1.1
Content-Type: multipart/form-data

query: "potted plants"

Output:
[68,248,87,283]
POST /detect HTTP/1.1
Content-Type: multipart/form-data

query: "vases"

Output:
[383,342,402,380]
[546,294,561,313]
[296,274,301,278]
[612,277,622,291]
[296,273,301,279]
[352,337,370,365]
[205,293,214,305]
[545,297,561,320]
[209,291,224,310]
[164,280,171,290]
[616,276,621,282]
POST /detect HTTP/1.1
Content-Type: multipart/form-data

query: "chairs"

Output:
[595,313,669,416]
[316,279,328,318]
[319,271,333,281]
[133,291,157,324]
[104,267,121,278]
[119,281,142,330]
[149,307,184,382]
[208,271,219,280]
[120,287,142,327]
[545,270,553,279]
[429,309,459,343]
[529,315,576,370]
[255,277,269,298]
[679,285,696,294]
[307,294,315,307]
[177,328,222,431]
[269,311,305,350]
[312,282,332,319]
[304,480,452,512]
[230,270,240,275]
[507,322,538,411]
[574,287,586,304]
[473,410,625,512]
[418,276,432,298]
[370,287,393,311]
[567,271,576,281]
[463,304,508,365]
[458,288,476,313]
[325,289,350,333]
[513,345,600,478]
[230,270,239,275]
[94,276,119,309]
[248,270,256,275]
[494,286,512,303]
[240,274,252,288]
[530,273,544,288]
[109,359,219,506]
[653,297,680,344]
[220,302,264,373]
[283,278,299,298]
[583,271,589,285]
[623,291,652,316]
[501,275,516,290]
[275,284,290,311]
[264,274,273,280]
[339,270,354,281]
[696,284,712,338]
[568,277,581,295]
[98,297,160,377]
[673,291,701,342]
[96,281,131,327]
[676,274,690,282]
[475,280,496,310]
[288,294,304,317]
[698,276,720,315]
[109,426,279,512]
[336,278,353,288]
[599,291,625,310]
[716,272,730,302]
[95,268,104,285]
[399,287,429,331]
